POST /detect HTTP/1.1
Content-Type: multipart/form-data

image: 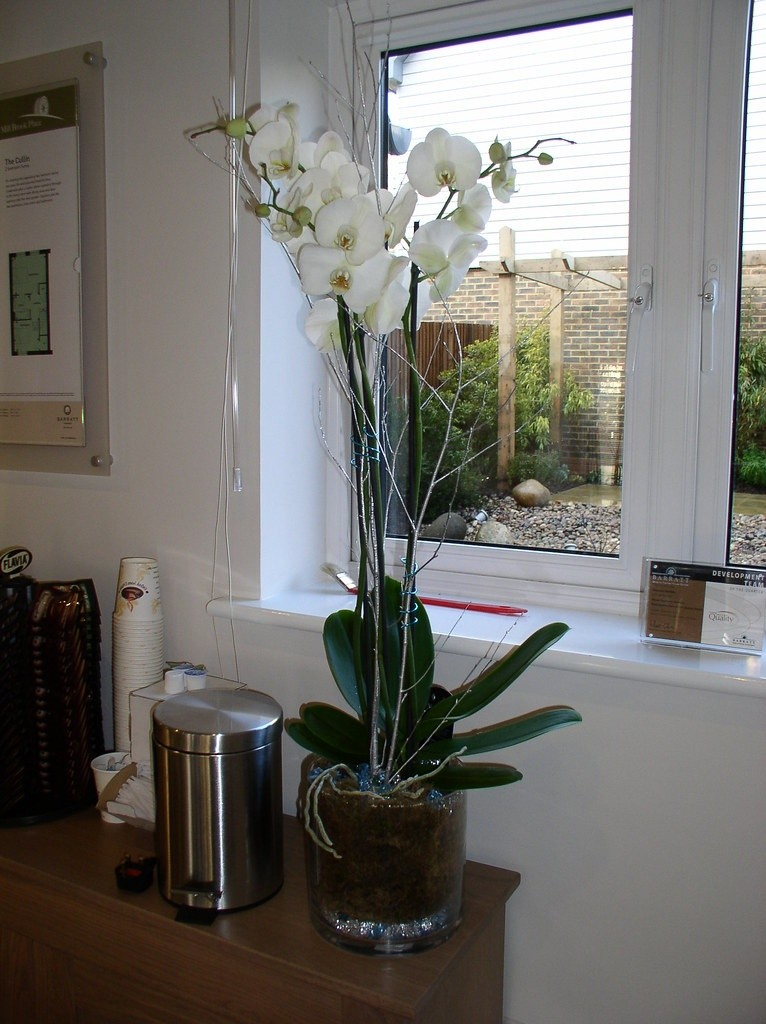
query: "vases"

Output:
[297,756,468,955]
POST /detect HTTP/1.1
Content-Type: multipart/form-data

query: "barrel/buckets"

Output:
[152,690,283,926]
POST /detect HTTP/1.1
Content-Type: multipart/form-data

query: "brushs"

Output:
[321,563,528,614]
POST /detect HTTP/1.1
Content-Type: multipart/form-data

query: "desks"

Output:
[0,802,522,1024]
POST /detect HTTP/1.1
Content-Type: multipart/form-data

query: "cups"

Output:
[164,670,184,694]
[112,557,165,752]
[90,752,131,824]
[173,666,207,691]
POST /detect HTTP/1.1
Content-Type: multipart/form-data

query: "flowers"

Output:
[208,104,582,794]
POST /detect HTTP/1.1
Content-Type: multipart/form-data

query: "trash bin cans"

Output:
[151,688,283,927]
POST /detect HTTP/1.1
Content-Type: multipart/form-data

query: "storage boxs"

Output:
[94,673,250,831]
